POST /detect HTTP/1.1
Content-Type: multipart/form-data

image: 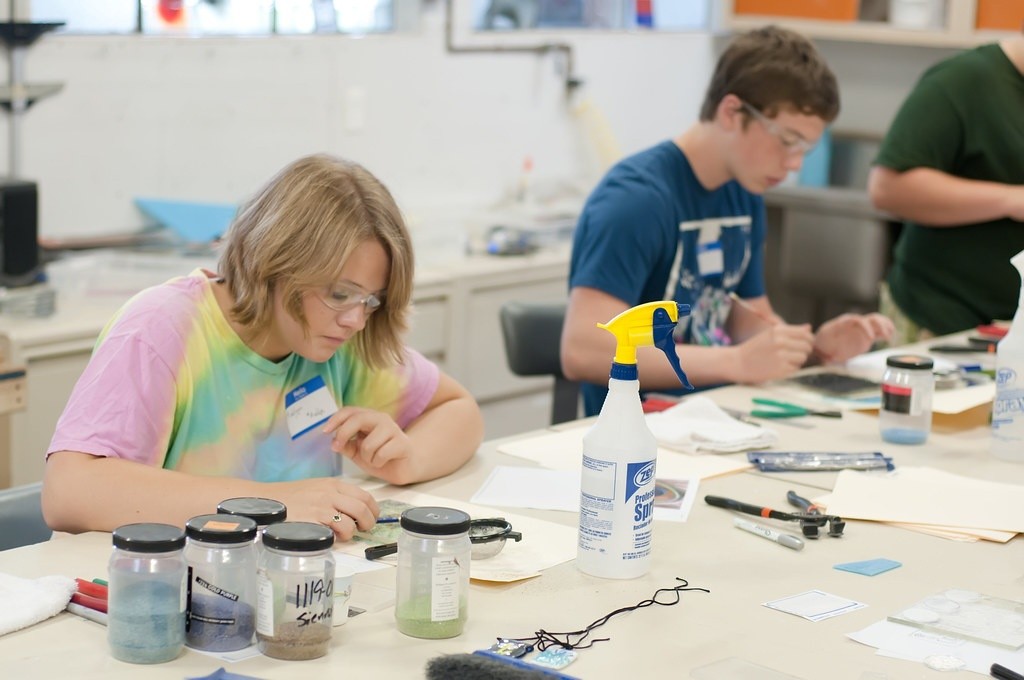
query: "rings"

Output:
[330,513,342,523]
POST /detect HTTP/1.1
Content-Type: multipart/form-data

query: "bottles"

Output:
[257,520,336,662]
[183,514,263,652]
[106,521,187,666]
[487,229,517,255]
[215,498,288,604]
[392,505,473,640]
[878,353,932,445]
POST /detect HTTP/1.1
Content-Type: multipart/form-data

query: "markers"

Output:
[372,519,399,523]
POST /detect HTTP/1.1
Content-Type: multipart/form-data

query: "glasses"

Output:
[310,279,389,314]
[738,100,821,157]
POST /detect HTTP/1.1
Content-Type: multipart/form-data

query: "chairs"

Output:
[498,302,584,425]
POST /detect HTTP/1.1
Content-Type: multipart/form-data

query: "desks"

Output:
[0,315,1024,680]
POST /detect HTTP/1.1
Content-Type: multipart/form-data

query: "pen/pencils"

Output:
[730,520,804,553]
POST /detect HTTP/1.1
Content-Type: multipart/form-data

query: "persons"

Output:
[558,25,895,418]
[866,20,1024,349]
[39,152,485,544]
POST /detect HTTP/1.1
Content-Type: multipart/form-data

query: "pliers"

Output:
[930,338,1000,352]
[750,398,844,418]
[704,489,846,539]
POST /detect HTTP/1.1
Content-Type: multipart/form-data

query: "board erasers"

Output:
[425,649,583,680]
